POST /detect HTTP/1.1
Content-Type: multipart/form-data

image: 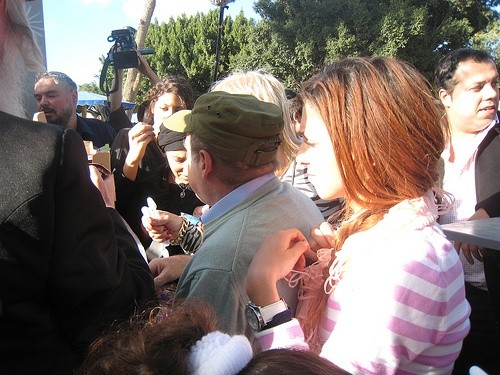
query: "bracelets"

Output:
[171,216,190,245]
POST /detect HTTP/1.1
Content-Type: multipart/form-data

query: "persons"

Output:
[423,47,500,342]
[36,47,426,299]
[244,55,473,375]
[82,300,353,375]
[0,1,162,375]
[140,91,331,358]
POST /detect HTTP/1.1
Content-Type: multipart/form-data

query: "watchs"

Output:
[244,297,289,332]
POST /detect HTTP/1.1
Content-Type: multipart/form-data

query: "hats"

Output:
[155,110,192,151]
[184,91,284,165]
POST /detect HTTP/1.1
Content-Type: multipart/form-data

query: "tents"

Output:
[75,92,136,107]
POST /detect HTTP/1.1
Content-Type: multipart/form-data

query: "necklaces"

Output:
[170,174,190,198]
